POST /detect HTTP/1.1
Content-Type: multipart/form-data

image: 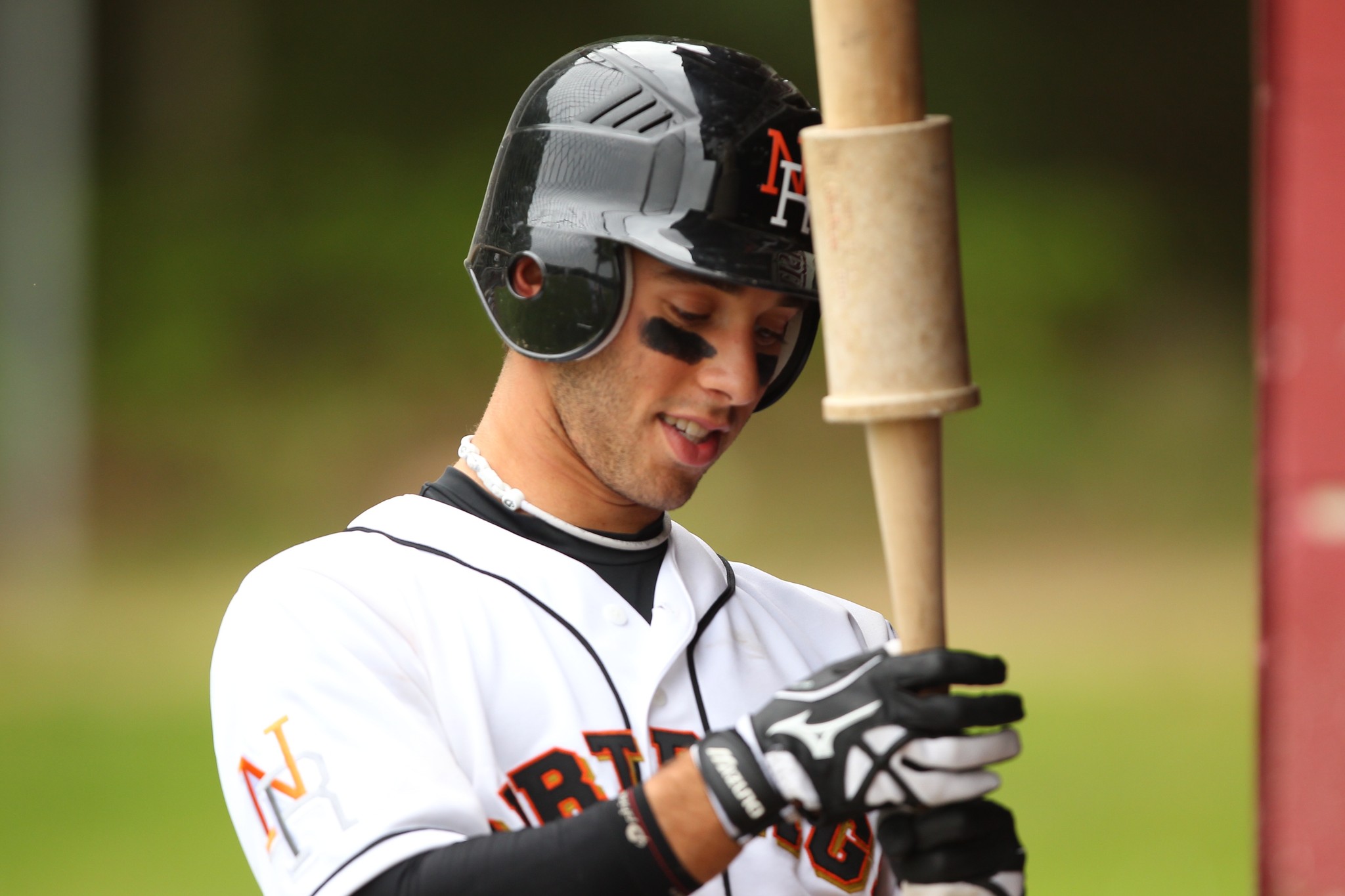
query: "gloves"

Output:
[689,639,1024,843]
[876,801,1026,896]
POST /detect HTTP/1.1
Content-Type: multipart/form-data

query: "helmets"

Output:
[463,35,820,412]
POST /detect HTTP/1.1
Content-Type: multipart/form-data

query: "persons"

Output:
[205,37,1031,896]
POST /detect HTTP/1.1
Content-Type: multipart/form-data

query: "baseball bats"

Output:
[798,0,983,693]
